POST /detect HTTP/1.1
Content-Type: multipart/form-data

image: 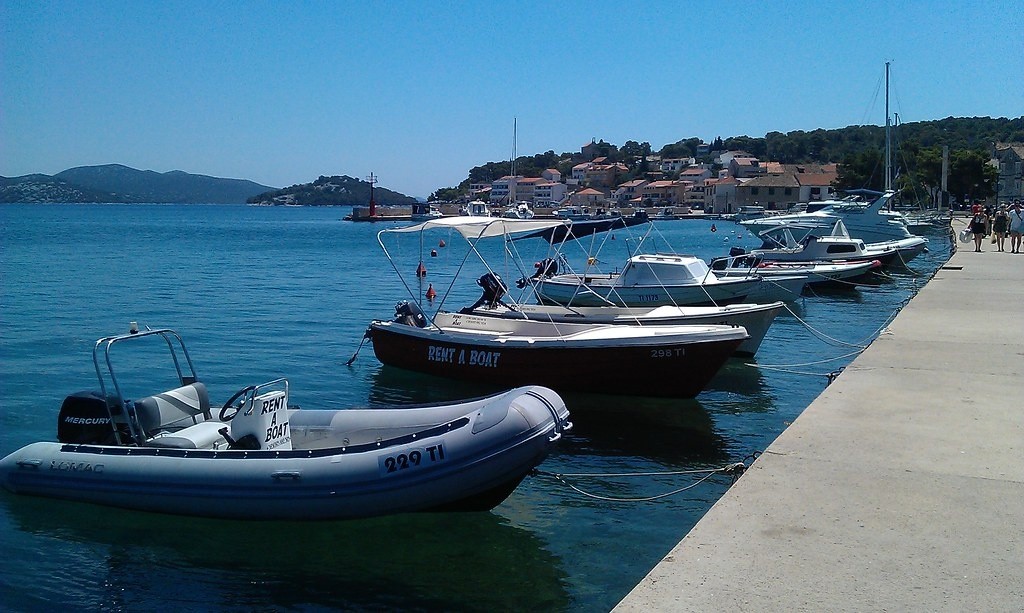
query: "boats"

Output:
[363,214,753,402]
[410,202,443,222]
[0,320,572,525]
[458,185,953,358]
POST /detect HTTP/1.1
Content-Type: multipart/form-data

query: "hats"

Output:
[1014,199,1021,204]
[999,204,1006,208]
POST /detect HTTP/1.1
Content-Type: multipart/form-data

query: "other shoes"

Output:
[1014,251,1019,254]
[974,250,977,252]
[1002,249,1004,251]
[998,250,1000,251]
[978,250,981,252]
[1011,250,1015,253]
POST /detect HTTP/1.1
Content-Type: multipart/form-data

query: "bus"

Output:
[737,205,765,215]
[793,203,808,211]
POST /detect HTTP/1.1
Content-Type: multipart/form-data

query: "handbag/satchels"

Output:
[991,230,996,244]
[959,228,973,244]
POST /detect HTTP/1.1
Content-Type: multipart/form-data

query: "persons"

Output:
[967,208,988,252]
[984,200,1024,254]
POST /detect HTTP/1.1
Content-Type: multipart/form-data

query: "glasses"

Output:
[978,211,983,212]
[1016,205,1019,207]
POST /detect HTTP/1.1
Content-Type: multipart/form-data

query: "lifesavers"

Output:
[958,229,973,244]
